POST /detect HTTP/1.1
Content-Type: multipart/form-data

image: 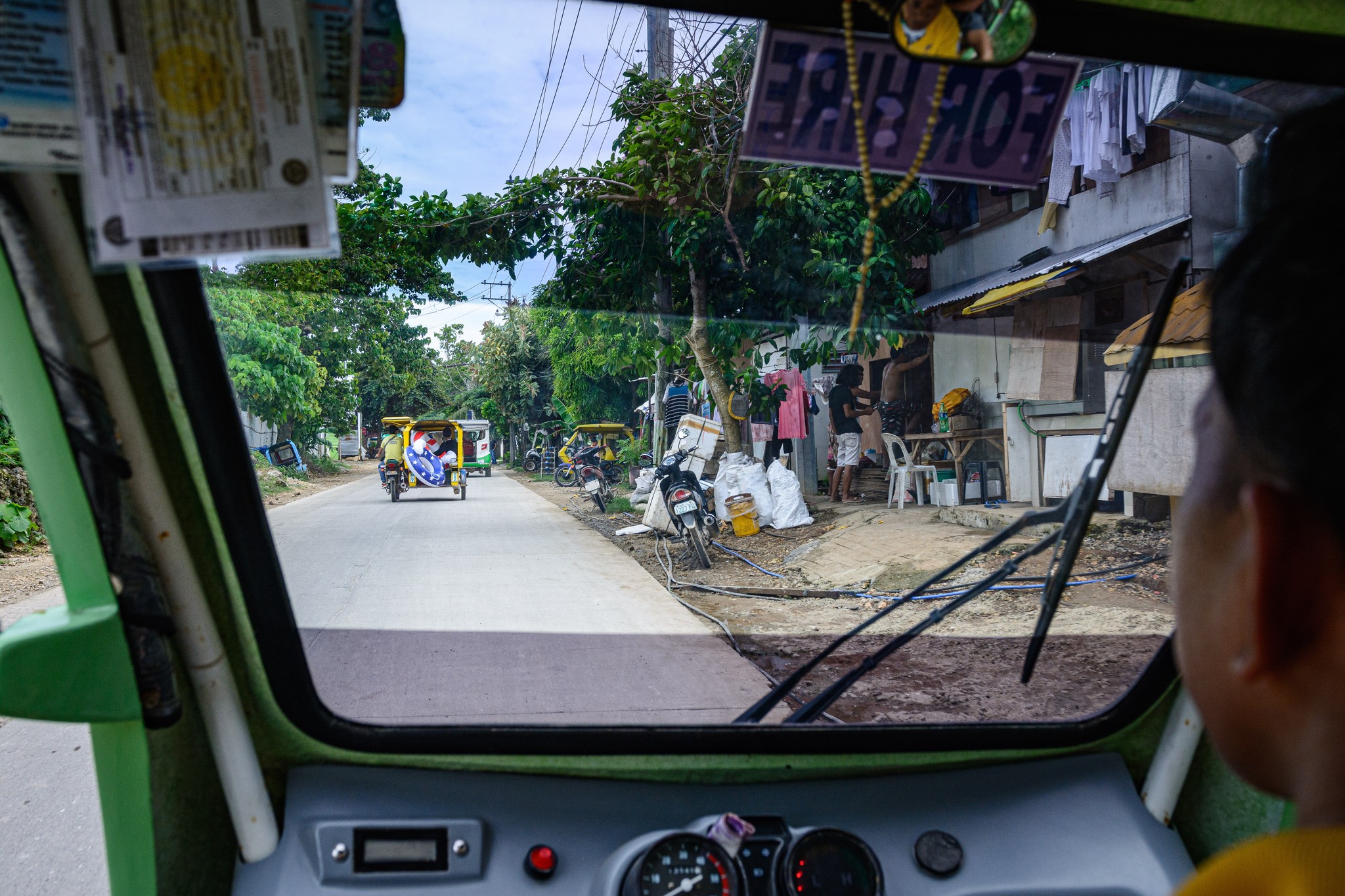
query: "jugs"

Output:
[864,449,876,463]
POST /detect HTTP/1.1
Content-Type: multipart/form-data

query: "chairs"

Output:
[881,433,941,509]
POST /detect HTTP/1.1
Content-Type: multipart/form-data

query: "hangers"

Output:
[674,373,685,386]
[783,362,794,371]
[769,364,780,373]
[1073,63,1124,90]
[802,381,817,396]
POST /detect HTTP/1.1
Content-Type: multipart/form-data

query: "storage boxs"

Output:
[670,413,722,461]
[930,480,1002,507]
[655,450,706,487]
[641,486,680,536]
[897,460,955,496]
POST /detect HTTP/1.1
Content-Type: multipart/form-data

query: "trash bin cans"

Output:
[628,465,640,485]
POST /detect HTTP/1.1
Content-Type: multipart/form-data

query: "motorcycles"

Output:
[638,428,724,570]
[555,444,626,487]
[448,420,492,478]
[558,424,635,474]
[522,443,549,473]
[374,417,468,503]
[563,435,614,514]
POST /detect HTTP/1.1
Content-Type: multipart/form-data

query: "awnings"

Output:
[905,211,1191,316]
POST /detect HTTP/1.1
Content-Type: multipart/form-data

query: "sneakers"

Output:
[404,481,410,486]
[382,482,388,489]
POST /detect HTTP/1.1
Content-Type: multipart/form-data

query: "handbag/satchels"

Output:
[379,453,385,461]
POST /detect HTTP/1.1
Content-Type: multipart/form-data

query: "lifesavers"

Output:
[404,430,457,488]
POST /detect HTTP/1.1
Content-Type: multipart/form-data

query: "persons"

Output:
[432,427,458,457]
[1164,89,1345,890]
[587,432,604,467]
[489,436,497,464]
[829,364,877,504]
[375,425,409,489]
[828,362,881,497]
[878,338,933,472]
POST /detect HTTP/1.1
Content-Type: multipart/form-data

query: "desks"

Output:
[905,426,1004,505]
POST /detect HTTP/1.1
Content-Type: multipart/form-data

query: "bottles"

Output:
[939,402,949,432]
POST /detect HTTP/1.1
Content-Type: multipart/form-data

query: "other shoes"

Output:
[494,463,497,464]
[491,464,494,464]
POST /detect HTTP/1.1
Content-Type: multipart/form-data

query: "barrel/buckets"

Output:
[725,500,760,537]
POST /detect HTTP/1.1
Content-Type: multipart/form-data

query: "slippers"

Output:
[829,497,842,502]
[842,497,862,504]
[983,500,1001,509]
[996,499,1013,504]
[838,491,859,497]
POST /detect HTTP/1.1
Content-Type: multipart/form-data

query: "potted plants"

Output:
[615,438,648,489]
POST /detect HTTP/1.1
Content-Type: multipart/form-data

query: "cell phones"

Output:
[873,406,878,410]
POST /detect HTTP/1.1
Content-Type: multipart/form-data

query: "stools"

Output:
[961,461,1007,503]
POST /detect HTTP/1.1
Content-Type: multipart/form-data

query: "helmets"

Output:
[591,437,598,441]
[587,433,594,436]
